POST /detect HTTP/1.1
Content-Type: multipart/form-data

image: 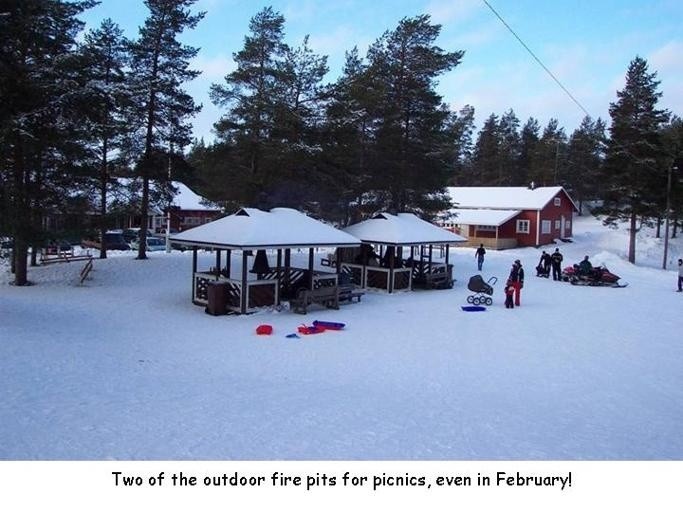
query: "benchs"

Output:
[290,284,364,316]
[424,271,457,290]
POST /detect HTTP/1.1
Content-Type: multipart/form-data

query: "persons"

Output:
[551,248,563,281]
[504,259,524,308]
[579,255,592,274]
[538,251,551,276]
[475,243,486,271]
[676,259,683,292]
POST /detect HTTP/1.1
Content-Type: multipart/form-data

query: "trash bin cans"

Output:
[205,281,226,316]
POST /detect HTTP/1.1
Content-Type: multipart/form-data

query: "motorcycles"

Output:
[562,262,630,288]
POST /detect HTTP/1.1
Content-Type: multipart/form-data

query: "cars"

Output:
[123,226,178,251]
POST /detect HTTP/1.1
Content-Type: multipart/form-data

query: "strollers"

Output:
[467,275,498,305]
